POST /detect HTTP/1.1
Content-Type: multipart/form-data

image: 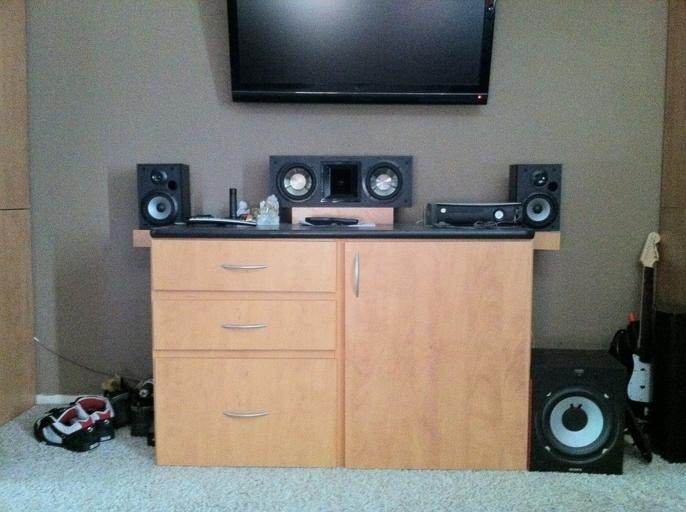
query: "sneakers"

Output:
[103,374,154,436]
[34,395,116,452]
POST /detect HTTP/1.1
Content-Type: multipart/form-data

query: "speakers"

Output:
[532,345,628,474]
[509,164,562,230]
[269,155,413,208]
[136,163,191,229]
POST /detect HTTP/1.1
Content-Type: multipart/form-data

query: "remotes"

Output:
[306,217,358,225]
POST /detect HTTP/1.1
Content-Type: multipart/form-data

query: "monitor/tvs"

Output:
[227,0,496,104]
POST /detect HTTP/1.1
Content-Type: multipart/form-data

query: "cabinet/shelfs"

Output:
[0,0,35,429]
[133,224,562,467]
[656,3,686,314]
